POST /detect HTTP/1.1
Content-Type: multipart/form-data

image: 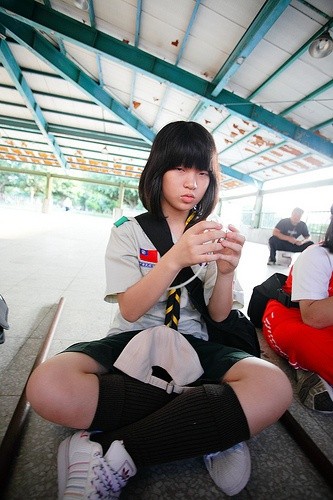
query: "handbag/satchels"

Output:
[191,281,261,359]
[245,273,300,329]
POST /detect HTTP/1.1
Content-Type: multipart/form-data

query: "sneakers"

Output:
[203,441,252,497]
[56,429,137,500]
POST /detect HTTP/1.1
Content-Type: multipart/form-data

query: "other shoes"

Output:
[296,368,333,414]
[267,259,276,266]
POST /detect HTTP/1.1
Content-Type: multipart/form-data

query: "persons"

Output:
[262,204,333,413]
[25,121,293,500]
[63,198,72,211]
